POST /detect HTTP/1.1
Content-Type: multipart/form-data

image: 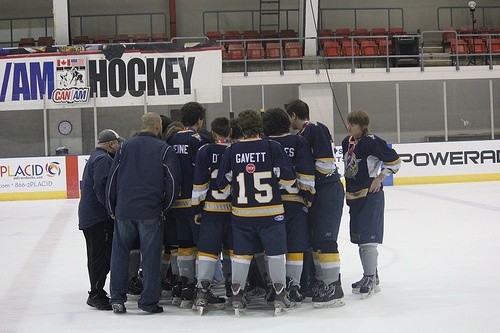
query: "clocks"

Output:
[58,120,72,135]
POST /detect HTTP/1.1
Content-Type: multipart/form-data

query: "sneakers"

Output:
[351,273,379,293]
[360,276,376,298]
[112,273,346,318]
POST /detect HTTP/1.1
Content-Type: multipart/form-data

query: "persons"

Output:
[77,98,346,310]
[106,112,183,315]
[341,109,402,294]
[216,109,297,310]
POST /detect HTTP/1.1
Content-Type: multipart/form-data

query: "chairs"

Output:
[440,25,500,53]
[19,33,168,48]
[320,27,405,57]
[207,29,304,60]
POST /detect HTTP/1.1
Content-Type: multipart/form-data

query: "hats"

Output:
[97,128,125,143]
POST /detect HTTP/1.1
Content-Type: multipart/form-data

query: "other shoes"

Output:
[87,290,113,310]
